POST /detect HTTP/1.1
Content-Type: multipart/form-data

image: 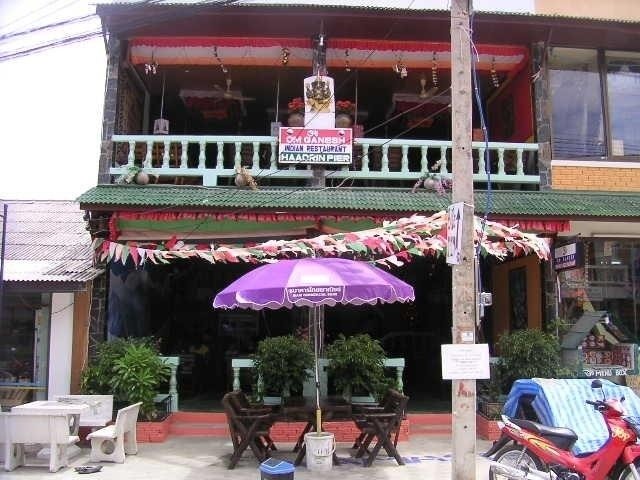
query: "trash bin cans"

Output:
[259,457,296,480]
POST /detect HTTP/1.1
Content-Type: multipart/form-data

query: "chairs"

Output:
[232,389,277,451]
[350,388,397,449]
[352,392,411,466]
[222,393,275,469]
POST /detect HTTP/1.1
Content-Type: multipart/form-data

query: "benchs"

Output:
[0,394,144,473]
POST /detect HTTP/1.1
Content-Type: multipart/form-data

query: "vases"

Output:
[288,113,304,126]
[335,113,352,128]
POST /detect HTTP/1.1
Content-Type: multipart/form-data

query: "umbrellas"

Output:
[211,256,418,407]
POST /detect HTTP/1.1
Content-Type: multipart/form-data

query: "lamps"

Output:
[153,67,170,135]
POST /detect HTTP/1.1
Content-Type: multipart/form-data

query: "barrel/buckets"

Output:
[303,431,338,473]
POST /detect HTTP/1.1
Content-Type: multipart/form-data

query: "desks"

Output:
[281,395,352,466]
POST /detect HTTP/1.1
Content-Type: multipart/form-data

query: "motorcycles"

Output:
[483,380,639,480]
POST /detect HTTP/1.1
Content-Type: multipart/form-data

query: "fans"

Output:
[395,78,446,105]
[210,78,256,104]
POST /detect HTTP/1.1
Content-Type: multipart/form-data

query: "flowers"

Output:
[335,100,354,116]
[287,97,304,114]
